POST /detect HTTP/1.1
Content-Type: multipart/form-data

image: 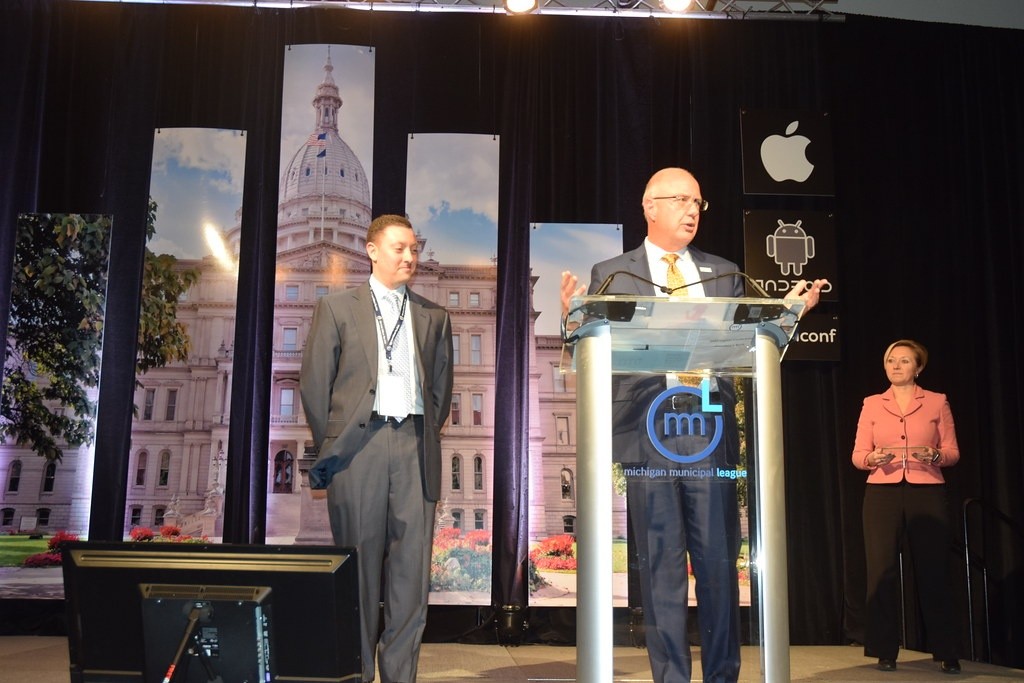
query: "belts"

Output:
[664,392,721,410]
[370,410,421,421]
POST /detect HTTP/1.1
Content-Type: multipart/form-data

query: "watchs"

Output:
[932,447,940,463]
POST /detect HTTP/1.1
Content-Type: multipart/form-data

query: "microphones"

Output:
[667,272,770,297]
[593,270,668,296]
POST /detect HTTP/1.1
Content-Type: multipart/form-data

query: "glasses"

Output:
[653,196,708,211]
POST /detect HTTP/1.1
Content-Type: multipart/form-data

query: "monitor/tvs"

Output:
[60,539,366,683]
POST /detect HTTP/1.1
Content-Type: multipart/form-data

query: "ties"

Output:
[663,252,703,387]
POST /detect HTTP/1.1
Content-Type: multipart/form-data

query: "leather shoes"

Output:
[878,657,896,671]
[941,658,960,674]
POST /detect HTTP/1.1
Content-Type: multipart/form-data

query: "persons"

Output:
[560,167,827,683]
[851,340,962,674]
[300,214,454,683]
